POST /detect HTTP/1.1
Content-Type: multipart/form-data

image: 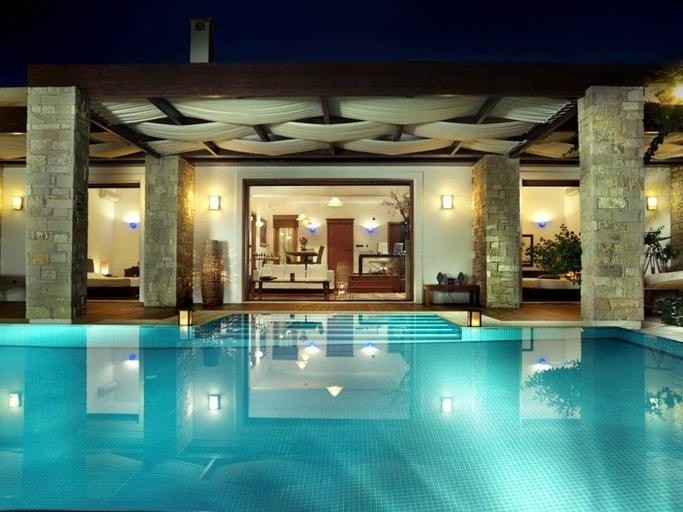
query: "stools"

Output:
[368,260,388,274]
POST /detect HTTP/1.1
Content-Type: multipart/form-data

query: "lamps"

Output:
[208,195,220,210]
[467,309,482,327]
[539,222,545,227]
[130,223,136,229]
[208,396,221,410]
[327,197,343,208]
[176,304,193,326]
[441,195,452,209]
[12,196,22,210]
[326,386,343,398]
[442,398,453,412]
[647,195,656,209]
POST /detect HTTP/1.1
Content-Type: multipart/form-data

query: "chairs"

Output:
[309,245,324,264]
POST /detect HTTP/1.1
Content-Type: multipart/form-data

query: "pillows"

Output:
[260,264,327,278]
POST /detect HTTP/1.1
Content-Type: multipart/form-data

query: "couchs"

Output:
[252,269,335,293]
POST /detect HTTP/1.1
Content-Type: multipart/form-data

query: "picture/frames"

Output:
[521,233,533,266]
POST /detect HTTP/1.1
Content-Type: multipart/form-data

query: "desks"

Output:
[359,253,405,276]
[423,284,480,307]
[288,250,318,264]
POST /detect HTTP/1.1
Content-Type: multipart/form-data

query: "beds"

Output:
[522,269,580,301]
[87,258,139,296]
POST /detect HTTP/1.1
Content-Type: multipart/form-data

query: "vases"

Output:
[301,241,306,251]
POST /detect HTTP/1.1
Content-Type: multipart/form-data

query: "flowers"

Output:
[299,235,308,251]
